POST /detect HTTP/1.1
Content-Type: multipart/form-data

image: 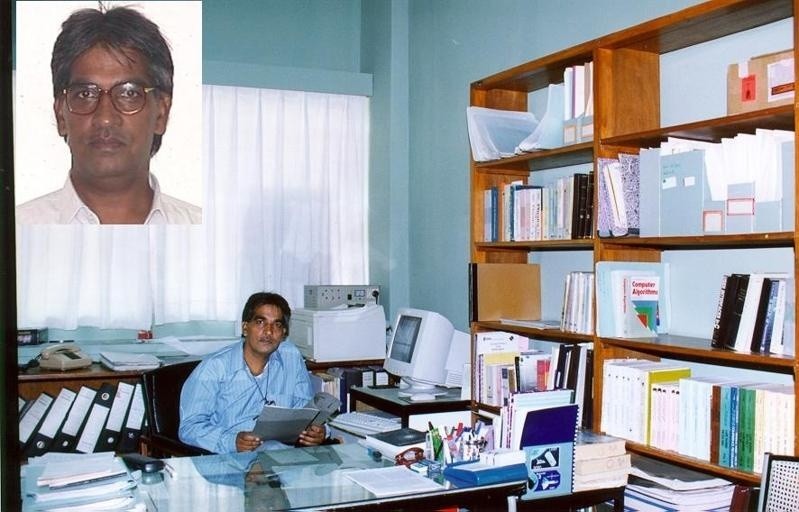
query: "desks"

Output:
[19,357,386,459]
[21,439,528,512]
[517,485,626,512]
[349,384,471,433]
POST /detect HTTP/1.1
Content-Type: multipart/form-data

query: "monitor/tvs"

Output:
[378,307,469,399]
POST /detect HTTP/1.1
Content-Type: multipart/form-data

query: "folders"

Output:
[118,383,147,452]
[51,385,97,453]
[31,388,78,456]
[28,455,149,512]
[355,366,375,387]
[72,382,117,453]
[18,395,28,418]
[18,392,56,454]
[368,366,389,387]
[343,368,361,412]
[95,382,136,453]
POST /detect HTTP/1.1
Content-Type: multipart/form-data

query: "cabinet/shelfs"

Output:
[469,0,799,485]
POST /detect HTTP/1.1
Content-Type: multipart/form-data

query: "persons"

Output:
[14,0,202,224]
[176,289,332,454]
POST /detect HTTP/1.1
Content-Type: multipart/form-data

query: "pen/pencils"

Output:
[429,422,480,463]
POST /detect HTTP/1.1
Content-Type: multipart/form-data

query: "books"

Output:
[19,450,147,512]
[250,391,343,443]
[99,352,165,374]
[471,269,799,512]
[360,428,427,457]
[483,169,594,243]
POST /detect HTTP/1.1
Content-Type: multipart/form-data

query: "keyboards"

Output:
[329,408,401,434]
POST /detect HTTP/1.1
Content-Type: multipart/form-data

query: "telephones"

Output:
[35,343,92,375]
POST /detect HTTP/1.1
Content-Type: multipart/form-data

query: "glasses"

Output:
[58,78,162,116]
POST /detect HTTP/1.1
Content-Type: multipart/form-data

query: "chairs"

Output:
[140,360,217,458]
